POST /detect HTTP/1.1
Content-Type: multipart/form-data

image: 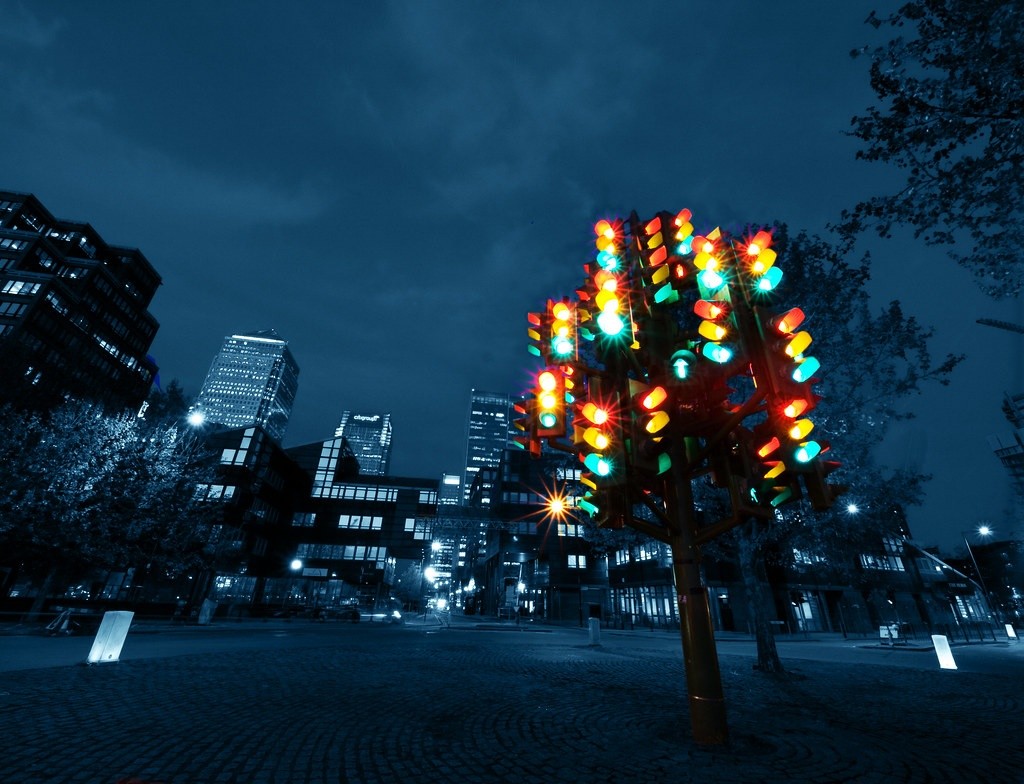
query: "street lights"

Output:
[962,523,1005,633]
[845,502,1004,633]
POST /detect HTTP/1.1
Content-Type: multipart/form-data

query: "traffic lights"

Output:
[514,205,848,534]
